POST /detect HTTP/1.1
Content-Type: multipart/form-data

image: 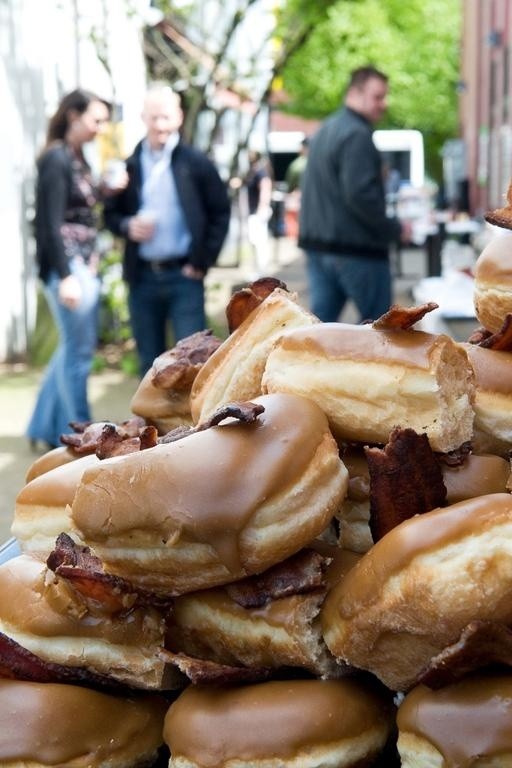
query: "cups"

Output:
[137,209,159,225]
[105,159,127,190]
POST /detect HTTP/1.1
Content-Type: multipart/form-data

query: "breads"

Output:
[0,183,512,768]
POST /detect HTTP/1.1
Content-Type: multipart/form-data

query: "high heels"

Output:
[29,438,57,456]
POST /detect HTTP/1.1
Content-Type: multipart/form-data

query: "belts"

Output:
[135,255,193,273]
